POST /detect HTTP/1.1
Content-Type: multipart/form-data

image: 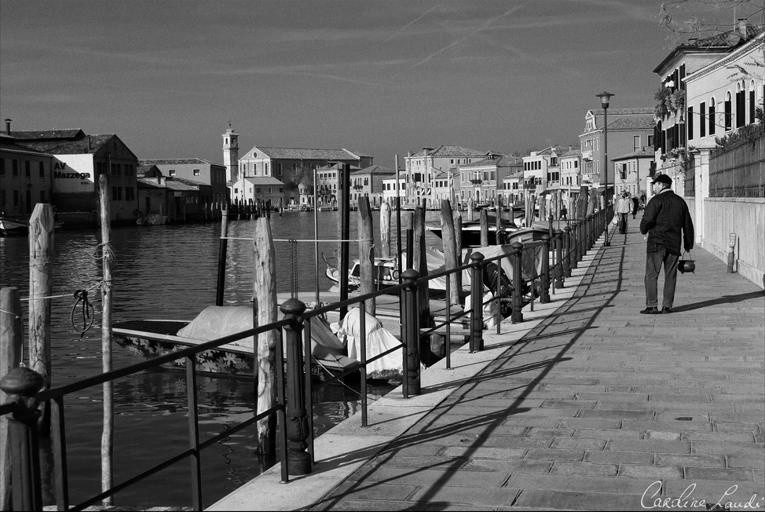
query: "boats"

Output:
[112,213,566,387]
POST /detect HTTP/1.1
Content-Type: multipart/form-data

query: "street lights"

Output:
[595,91,615,246]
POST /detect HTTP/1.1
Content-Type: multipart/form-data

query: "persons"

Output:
[615,192,646,234]
[639,173,694,313]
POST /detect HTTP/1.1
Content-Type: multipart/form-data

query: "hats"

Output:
[650,174,672,184]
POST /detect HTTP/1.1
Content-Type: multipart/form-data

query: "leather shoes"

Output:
[661,306,671,313]
[640,306,658,314]
